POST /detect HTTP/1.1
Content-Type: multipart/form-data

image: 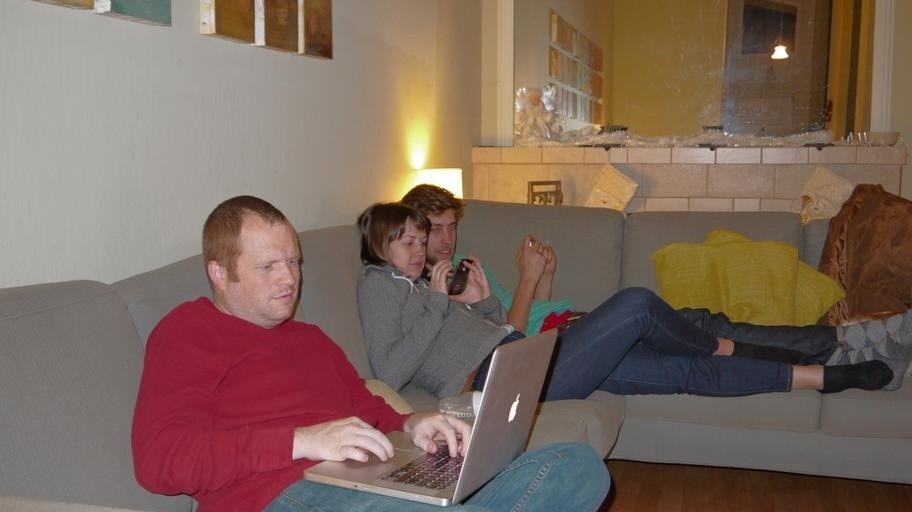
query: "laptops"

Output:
[304,328,559,507]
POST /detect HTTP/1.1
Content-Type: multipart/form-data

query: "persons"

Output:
[355,201,894,398]
[401,182,841,346]
[128,193,612,512]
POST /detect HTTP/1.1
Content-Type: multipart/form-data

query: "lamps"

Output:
[415,167,464,200]
[771,0,789,59]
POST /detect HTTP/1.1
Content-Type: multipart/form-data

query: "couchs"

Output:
[0,199,912,512]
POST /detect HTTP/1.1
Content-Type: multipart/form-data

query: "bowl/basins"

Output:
[866,131,900,146]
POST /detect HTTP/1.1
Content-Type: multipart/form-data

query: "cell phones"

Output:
[449,259,473,294]
